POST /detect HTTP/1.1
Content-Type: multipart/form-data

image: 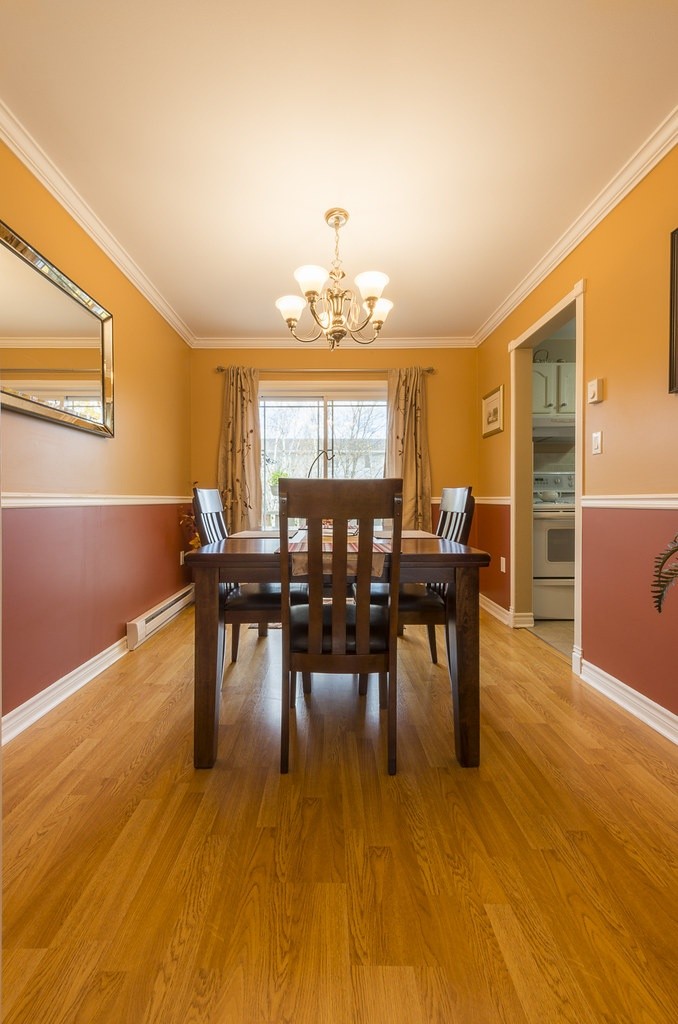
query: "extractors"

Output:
[533,428,574,443]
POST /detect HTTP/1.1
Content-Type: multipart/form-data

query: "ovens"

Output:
[532,503,575,620]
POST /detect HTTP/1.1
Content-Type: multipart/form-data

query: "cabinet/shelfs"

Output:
[532,362,576,415]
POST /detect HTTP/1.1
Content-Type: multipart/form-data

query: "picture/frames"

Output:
[481,384,504,439]
[668,228,678,395]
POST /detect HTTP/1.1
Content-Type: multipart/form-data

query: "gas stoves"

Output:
[533,471,575,504]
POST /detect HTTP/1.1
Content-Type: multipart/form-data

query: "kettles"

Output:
[536,489,562,502]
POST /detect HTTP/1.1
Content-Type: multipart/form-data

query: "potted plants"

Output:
[268,470,289,496]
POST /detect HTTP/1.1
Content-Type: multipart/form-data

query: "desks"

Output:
[184,525,491,770]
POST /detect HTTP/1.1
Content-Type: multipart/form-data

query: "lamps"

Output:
[275,207,394,352]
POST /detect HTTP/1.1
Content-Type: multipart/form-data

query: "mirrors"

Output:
[0,219,115,440]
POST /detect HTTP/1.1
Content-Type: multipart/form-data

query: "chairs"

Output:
[351,486,476,695]
[191,486,312,695]
[279,478,405,775]
